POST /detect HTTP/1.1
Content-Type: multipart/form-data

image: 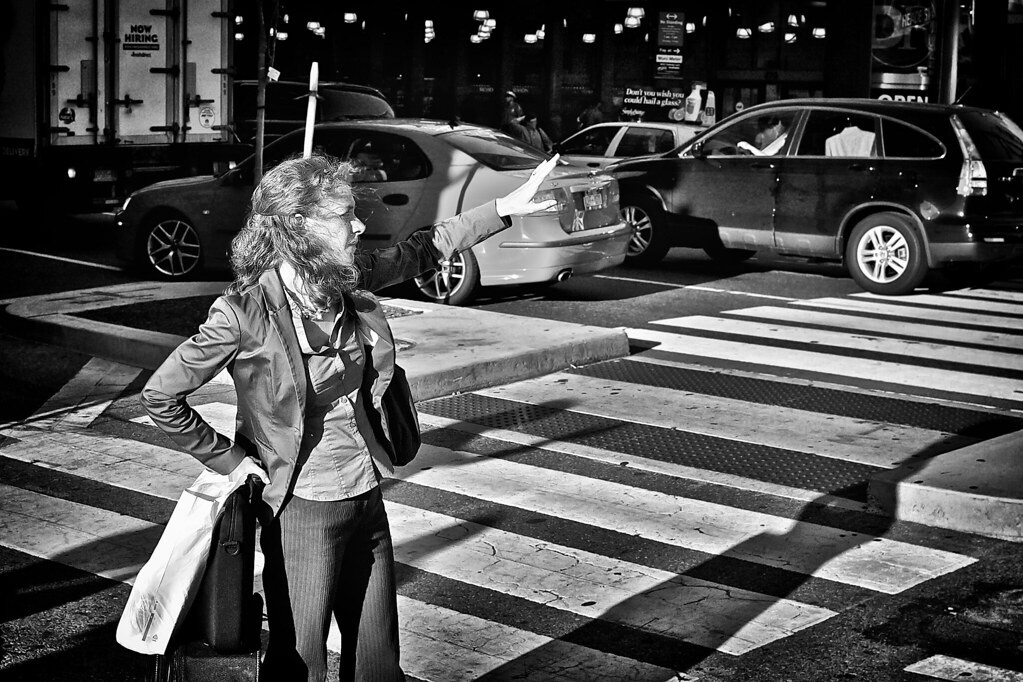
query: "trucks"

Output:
[0,0,241,211]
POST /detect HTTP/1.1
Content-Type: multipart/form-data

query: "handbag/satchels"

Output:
[115,458,253,655]
[342,291,421,466]
[204,481,255,652]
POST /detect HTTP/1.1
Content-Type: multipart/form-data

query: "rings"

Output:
[533,172,536,175]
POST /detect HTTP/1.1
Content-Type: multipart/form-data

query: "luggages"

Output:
[150,474,262,682]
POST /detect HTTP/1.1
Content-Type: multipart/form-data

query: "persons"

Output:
[737,130,790,157]
[139,153,560,682]
[510,112,552,152]
[504,90,522,135]
[578,96,607,146]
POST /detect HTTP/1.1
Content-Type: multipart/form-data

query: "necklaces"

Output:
[283,284,331,321]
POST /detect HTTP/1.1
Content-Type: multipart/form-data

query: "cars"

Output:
[112,116,634,306]
[545,121,708,170]
[231,79,395,129]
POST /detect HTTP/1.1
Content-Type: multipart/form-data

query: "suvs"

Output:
[605,96,1022,296]
